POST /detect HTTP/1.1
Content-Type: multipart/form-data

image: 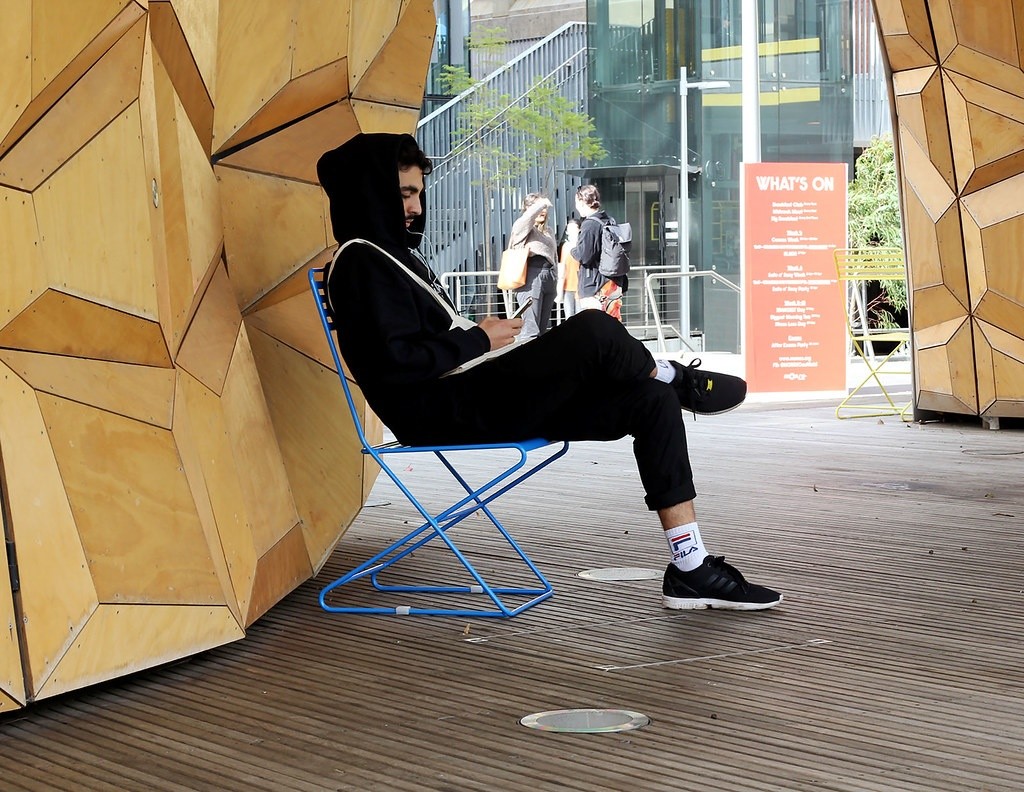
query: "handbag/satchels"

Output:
[497,235,531,290]
[553,263,566,304]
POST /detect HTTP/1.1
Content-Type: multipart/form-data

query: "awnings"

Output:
[556,162,703,179]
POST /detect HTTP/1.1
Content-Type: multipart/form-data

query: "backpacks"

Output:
[587,214,633,276]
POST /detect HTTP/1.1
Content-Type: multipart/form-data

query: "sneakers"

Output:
[668,357,747,421]
[662,554,783,610]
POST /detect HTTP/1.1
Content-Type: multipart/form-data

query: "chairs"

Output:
[832,247,915,422]
[307,268,569,619]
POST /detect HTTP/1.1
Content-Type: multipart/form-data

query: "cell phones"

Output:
[509,297,534,320]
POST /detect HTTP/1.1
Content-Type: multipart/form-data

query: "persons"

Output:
[570,183,629,322]
[509,193,558,336]
[316,130,785,610]
[553,217,586,320]
